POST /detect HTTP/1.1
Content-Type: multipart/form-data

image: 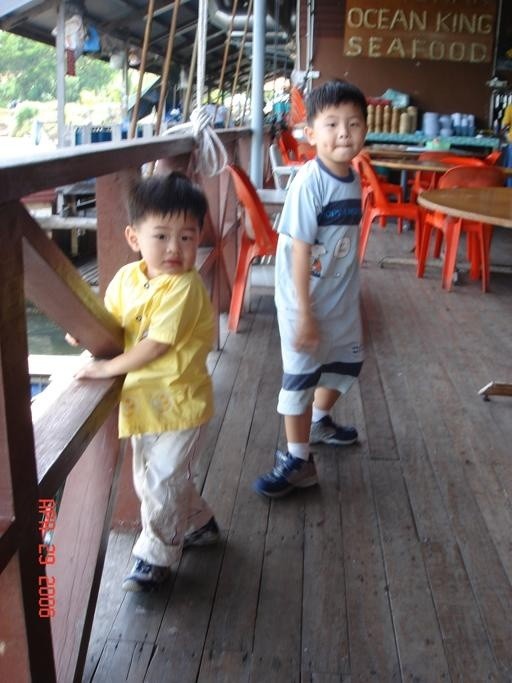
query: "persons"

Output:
[254,78,369,500]
[501,104,512,187]
[63,173,220,593]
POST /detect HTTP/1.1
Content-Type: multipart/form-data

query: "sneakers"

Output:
[307,413,360,449]
[177,512,224,552]
[251,446,320,499]
[119,556,174,593]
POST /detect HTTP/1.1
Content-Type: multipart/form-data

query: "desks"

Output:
[416,186,512,403]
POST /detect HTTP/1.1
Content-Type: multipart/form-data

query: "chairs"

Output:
[226,162,281,332]
[257,86,512,292]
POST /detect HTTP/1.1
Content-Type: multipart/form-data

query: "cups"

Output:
[422,110,474,135]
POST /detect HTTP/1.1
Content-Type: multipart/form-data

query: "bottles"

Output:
[365,102,418,134]
